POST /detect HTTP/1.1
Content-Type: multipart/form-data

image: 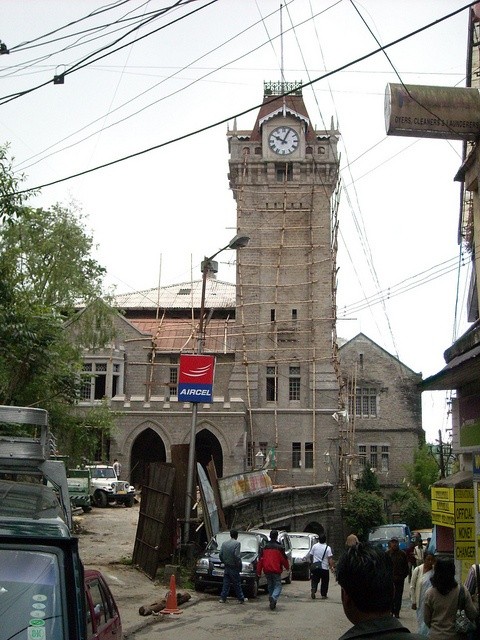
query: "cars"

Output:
[411,529,433,547]
[194,531,268,598]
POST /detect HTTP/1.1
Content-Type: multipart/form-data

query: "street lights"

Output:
[184,234,251,547]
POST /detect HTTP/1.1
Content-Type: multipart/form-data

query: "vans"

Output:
[287,532,320,581]
[247,527,292,585]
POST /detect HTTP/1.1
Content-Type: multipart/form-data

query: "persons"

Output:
[424,557,478,640]
[410,552,436,635]
[412,539,424,566]
[381,538,412,619]
[410,532,422,543]
[219,530,249,604]
[112,458,122,480]
[345,531,361,548]
[257,530,289,610]
[465,564,480,604]
[310,534,335,599]
[335,544,428,640]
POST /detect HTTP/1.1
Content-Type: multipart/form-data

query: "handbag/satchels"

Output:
[455,583,477,634]
[310,561,322,575]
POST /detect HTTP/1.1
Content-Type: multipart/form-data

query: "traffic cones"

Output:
[160,573,183,614]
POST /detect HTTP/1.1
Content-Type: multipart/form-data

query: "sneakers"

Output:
[219,599,229,603]
[322,596,329,599]
[268,596,275,610]
[395,614,399,618]
[311,589,316,599]
[241,597,248,604]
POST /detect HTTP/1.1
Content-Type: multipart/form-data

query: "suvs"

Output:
[46,469,92,515]
[75,464,136,507]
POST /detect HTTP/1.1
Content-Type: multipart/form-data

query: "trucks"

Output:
[366,523,413,558]
[0,480,123,639]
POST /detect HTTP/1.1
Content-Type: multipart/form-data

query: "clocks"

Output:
[268,126,299,156]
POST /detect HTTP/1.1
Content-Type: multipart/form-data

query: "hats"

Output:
[390,537,399,542]
